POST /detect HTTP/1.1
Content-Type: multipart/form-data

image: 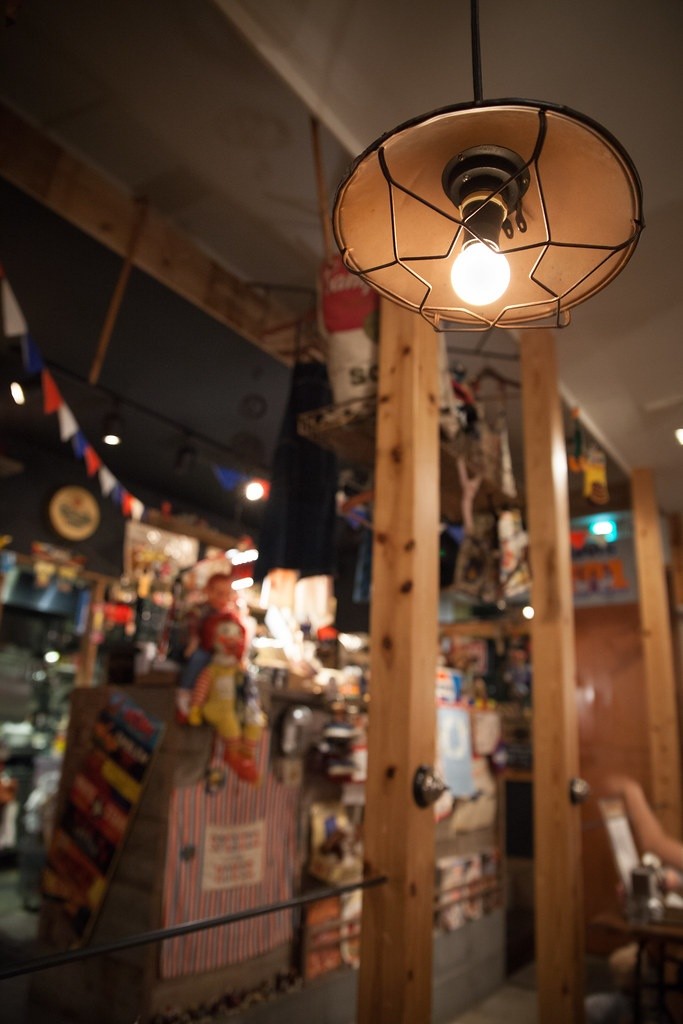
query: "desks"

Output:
[597,902,683,1024]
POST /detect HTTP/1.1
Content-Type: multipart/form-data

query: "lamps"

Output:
[331,0,646,333]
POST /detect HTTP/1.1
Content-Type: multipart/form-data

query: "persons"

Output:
[602,772,683,872]
